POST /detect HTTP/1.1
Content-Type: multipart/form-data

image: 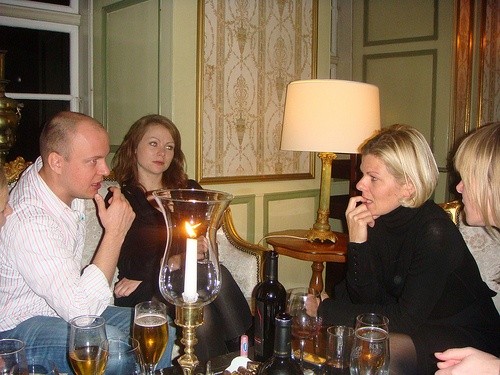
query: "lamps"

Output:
[280,79,384,244]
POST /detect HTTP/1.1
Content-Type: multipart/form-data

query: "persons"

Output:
[0,162,13,231]
[111,113,252,359]
[0,111,175,375]
[307,122,500,375]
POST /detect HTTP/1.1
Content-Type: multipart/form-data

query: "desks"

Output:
[267,230,352,297]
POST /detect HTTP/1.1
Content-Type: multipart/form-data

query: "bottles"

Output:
[258,312,303,375]
[254,250,288,361]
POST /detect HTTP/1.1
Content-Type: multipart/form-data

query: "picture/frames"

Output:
[194,0,320,183]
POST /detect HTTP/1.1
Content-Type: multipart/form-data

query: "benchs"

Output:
[2,156,266,368]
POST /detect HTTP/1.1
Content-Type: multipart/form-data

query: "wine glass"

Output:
[285,287,320,375]
[131,300,169,375]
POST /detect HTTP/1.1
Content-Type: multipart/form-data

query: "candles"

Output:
[183,221,200,300]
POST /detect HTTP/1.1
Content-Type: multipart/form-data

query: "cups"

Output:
[69,315,111,375]
[0,338,28,375]
[350,326,391,375]
[354,312,389,333]
[101,334,141,375]
[9,356,60,375]
[326,325,354,375]
